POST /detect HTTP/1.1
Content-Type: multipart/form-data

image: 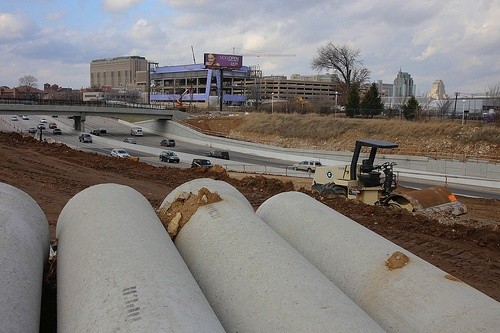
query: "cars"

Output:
[191,158,213,167]
[37,124,45,129]
[206,150,230,161]
[160,151,180,163]
[161,139,176,147]
[29,128,37,133]
[53,128,62,135]
[40,119,57,128]
[293,160,322,173]
[12,114,29,121]
[89,127,108,136]
[78,134,93,143]
[111,148,131,157]
[130,127,143,136]
[124,137,135,144]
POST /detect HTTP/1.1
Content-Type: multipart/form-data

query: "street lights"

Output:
[147,61,157,107]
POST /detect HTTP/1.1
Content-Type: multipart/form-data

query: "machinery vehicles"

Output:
[308,138,469,218]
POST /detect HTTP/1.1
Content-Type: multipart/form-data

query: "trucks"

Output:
[83,92,105,102]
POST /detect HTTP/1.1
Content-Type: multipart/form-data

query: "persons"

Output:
[205,54,220,66]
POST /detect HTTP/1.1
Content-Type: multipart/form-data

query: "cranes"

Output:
[220,47,297,57]
